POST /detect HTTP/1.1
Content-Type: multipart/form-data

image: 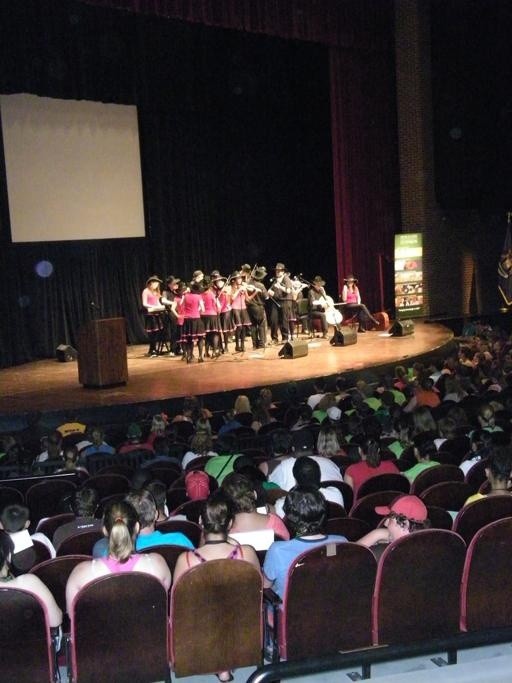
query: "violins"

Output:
[220,285,242,294]
[290,281,308,289]
[276,275,285,289]
[238,282,263,294]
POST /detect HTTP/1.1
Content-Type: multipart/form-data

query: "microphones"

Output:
[91,302,100,310]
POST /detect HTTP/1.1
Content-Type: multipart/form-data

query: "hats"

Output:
[292,431,314,449]
[313,275,326,286]
[343,275,359,283]
[375,493,428,523]
[184,470,209,499]
[125,422,142,434]
[145,263,286,294]
[325,405,342,420]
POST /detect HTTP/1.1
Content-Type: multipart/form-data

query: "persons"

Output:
[2,328,512,682]
[141,263,379,363]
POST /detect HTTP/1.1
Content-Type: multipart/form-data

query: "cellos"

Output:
[318,288,343,326]
[365,312,390,331]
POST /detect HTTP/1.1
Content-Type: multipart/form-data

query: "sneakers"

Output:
[321,334,330,340]
[150,346,264,363]
[269,339,289,345]
[356,321,380,331]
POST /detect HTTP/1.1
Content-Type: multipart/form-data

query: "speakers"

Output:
[393,319,414,336]
[56,343,78,362]
[330,327,357,346]
[278,339,308,359]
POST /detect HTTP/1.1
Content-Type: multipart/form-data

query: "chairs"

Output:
[297,299,322,338]
[339,295,356,329]
